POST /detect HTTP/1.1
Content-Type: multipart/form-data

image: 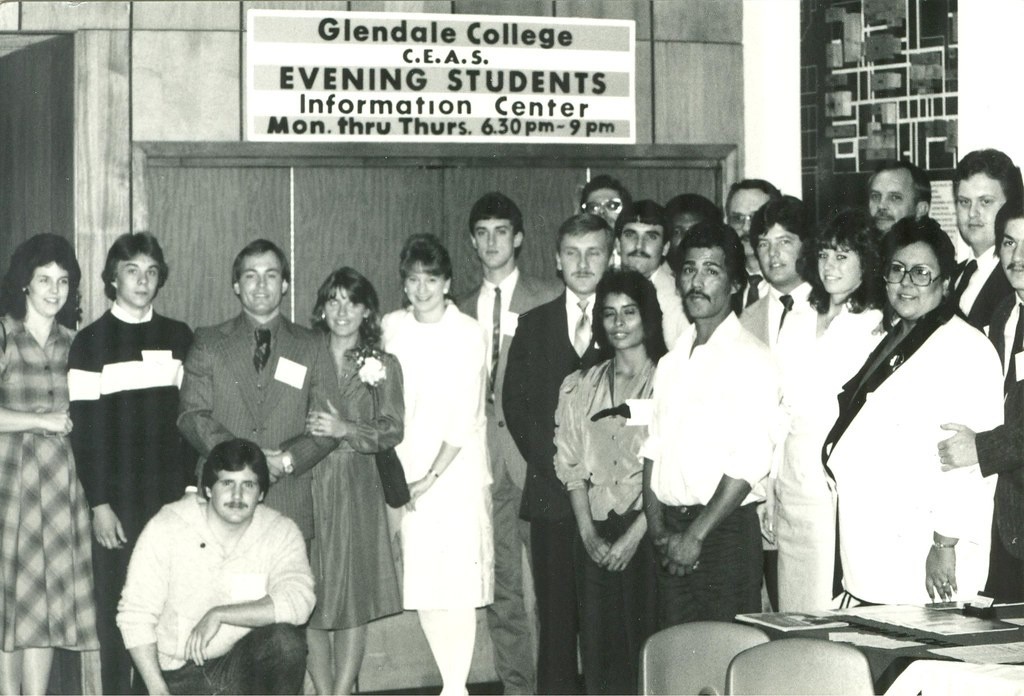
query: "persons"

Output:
[176,240,344,564]
[462,151,1024,696]
[115,437,317,696]
[376,233,487,696]
[66,231,195,696]
[303,265,404,696]
[0,232,101,696]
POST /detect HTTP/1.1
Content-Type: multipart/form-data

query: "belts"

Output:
[663,502,757,520]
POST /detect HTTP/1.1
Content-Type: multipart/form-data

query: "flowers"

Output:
[358,356,387,389]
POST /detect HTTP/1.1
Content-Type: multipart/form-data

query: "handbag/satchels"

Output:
[370,353,410,509]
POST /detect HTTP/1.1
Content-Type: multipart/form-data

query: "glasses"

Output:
[582,198,623,215]
[729,212,756,230]
[882,259,942,287]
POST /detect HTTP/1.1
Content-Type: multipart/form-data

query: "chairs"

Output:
[724,639,876,696]
[637,620,771,696]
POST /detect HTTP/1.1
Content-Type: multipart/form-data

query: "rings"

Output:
[942,581,950,586]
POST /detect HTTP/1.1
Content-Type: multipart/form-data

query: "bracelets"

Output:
[935,543,954,548]
[429,470,439,478]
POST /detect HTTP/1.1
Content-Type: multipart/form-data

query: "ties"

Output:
[1003,303,1024,398]
[746,275,762,308]
[954,260,977,310]
[490,287,500,391]
[253,329,271,375]
[779,294,794,330]
[575,300,591,359]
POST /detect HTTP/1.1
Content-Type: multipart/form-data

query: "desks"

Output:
[733,595,1024,696]
